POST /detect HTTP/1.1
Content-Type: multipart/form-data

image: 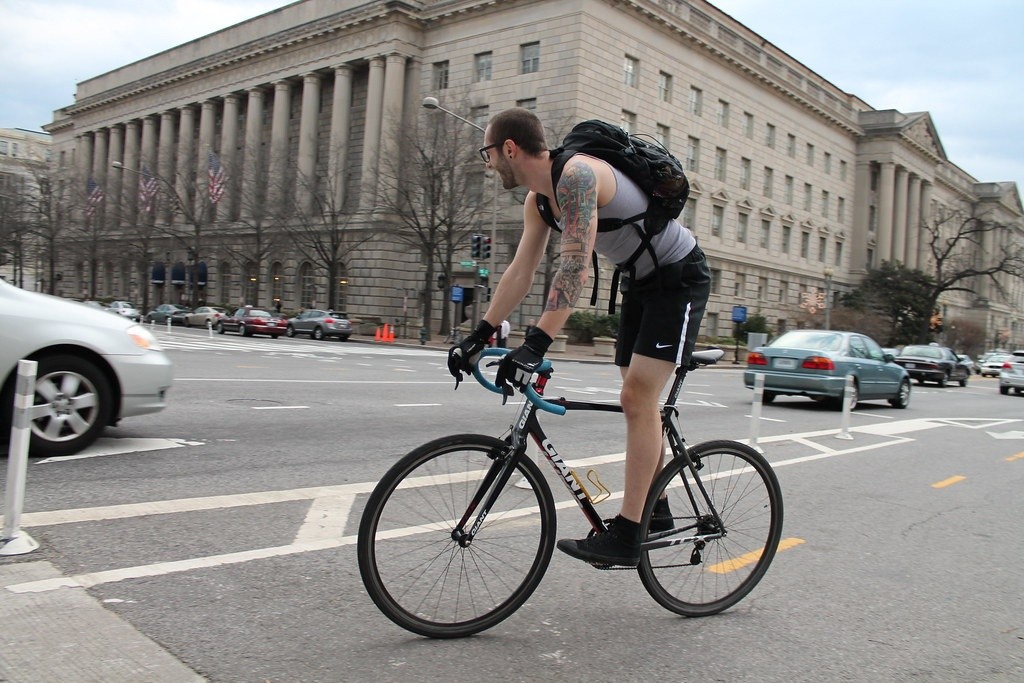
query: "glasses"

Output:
[479,143,497,163]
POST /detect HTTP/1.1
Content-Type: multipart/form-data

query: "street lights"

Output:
[823,264,837,331]
[422,97,498,303]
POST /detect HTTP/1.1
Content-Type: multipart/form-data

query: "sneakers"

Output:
[647,495,676,533]
[557,515,641,567]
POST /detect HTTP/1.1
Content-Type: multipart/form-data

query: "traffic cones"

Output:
[373,324,396,342]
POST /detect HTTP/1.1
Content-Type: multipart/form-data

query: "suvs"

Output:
[1000,349,1024,395]
[287,308,353,341]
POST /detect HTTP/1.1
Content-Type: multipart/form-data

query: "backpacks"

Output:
[536,119,690,237]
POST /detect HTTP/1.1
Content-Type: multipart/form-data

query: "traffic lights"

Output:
[482,236,492,259]
[471,235,481,258]
[482,287,491,302]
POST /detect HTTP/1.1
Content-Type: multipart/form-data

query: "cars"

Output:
[893,344,972,386]
[184,306,230,329]
[975,352,1014,377]
[145,303,193,324]
[0,277,172,457]
[957,354,975,372]
[744,327,910,409]
[215,304,288,338]
[105,300,143,320]
[881,348,899,358]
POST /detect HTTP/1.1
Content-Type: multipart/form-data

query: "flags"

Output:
[207,150,228,204]
[140,163,159,212]
[85,176,104,215]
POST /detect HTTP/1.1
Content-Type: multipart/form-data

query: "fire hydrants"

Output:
[419,328,427,345]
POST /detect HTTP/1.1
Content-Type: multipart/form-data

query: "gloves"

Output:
[448,320,495,382]
[495,325,554,393]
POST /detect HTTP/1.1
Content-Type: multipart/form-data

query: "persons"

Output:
[448,107,711,567]
[526,317,535,337]
[497,317,510,348]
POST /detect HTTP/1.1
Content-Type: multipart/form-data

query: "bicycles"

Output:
[357,348,785,640]
